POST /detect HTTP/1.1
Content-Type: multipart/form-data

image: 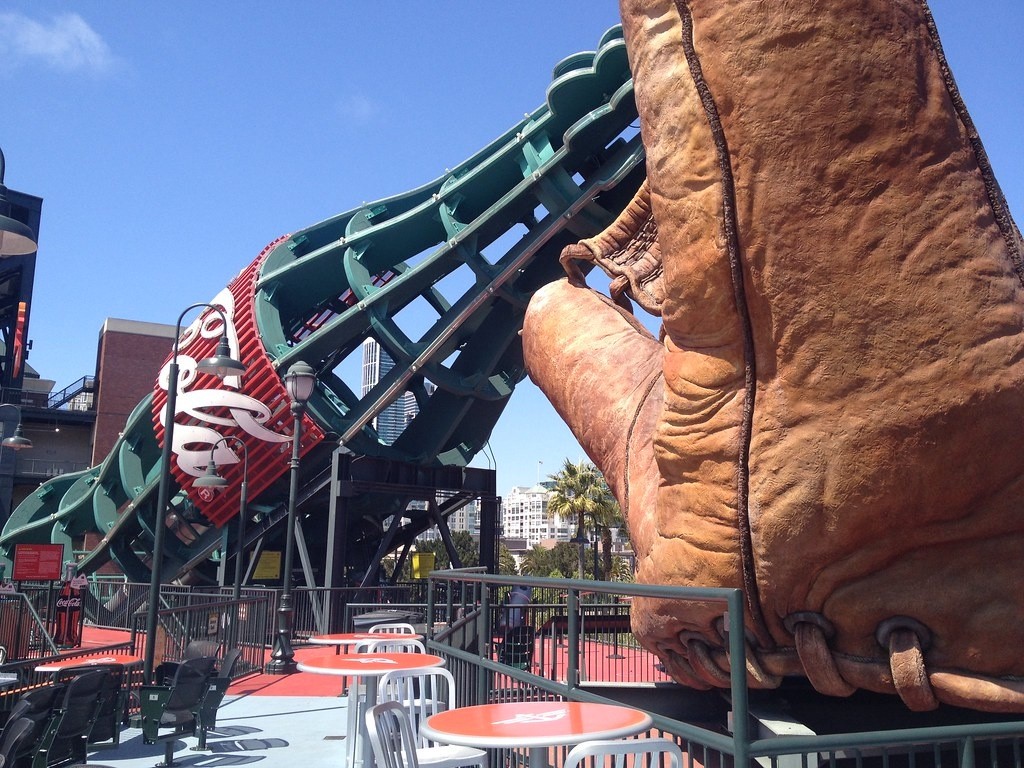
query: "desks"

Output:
[34,655,140,726]
[419,702,653,768]
[308,633,424,698]
[296,653,446,768]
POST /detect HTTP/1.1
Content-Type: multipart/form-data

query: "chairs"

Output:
[492,626,536,691]
[364,701,418,768]
[346,623,425,768]
[563,738,684,768]
[0,640,243,768]
[379,667,488,768]
